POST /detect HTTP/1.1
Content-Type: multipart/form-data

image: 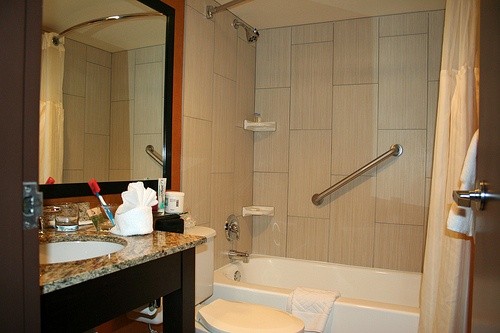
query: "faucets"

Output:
[38,216,44,238]
[228,250,249,264]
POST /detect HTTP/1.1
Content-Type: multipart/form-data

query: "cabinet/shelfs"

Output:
[243,120,276,217]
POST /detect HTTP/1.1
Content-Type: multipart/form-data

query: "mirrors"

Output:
[38,0,175,199]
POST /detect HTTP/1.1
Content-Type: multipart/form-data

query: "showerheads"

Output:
[233,18,258,43]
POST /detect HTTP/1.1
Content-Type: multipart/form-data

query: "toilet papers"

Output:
[131,300,158,316]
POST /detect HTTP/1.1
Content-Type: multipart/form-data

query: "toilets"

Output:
[183,226,306,333]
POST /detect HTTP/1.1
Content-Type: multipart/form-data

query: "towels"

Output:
[287,286,341,333]
[446,128,479,237]
[109,181,159,236]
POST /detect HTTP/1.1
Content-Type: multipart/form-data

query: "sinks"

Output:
[39,240,125,264]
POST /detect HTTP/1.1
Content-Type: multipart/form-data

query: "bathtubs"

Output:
[200,253,422,333]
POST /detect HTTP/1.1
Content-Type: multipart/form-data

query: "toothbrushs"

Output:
[88,178,116,226]
[45,177,55,184]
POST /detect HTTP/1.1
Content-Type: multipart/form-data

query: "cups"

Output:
[55,203,78,231]
[41,206,61,234]
[164,192,185,214]
[99,206,118,233]
